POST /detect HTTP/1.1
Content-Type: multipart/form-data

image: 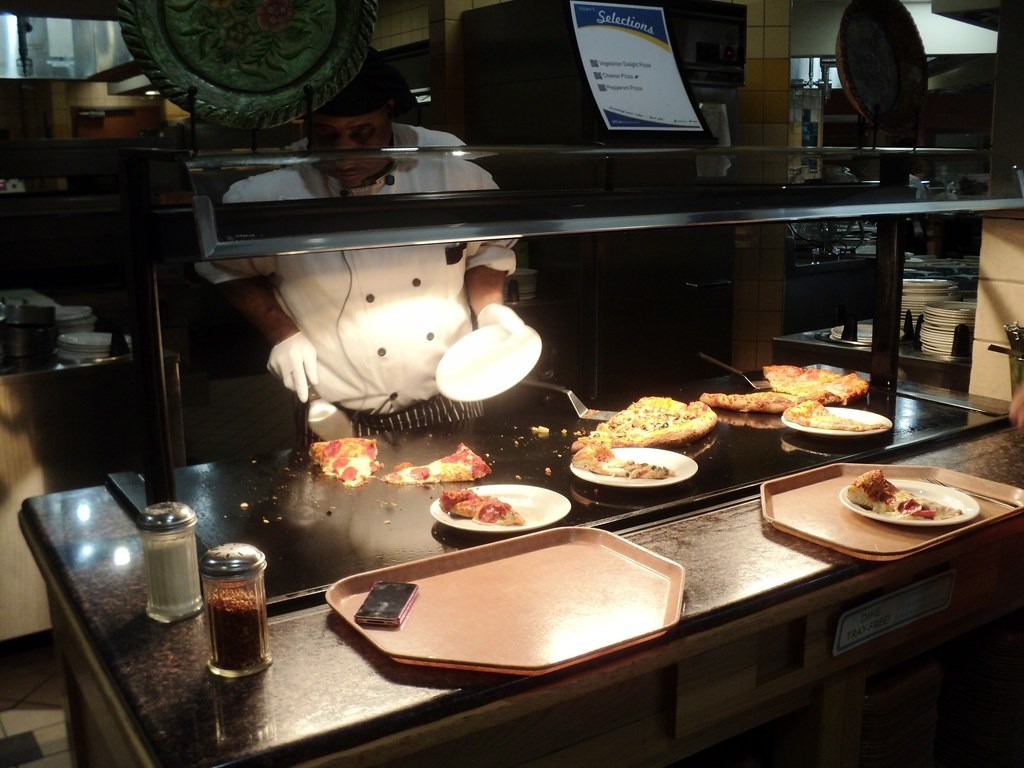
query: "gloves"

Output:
[477,302,526,335]
[265,333,318,403]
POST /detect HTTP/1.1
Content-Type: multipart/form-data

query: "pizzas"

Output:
[699,391,810,414]
[308,437,380,488]
[573,444,674,479]
[783,399,891,431]
[438,488,525,526]
[846,469,964,519]
[762,364,869,406]
[381,443,492,485]
[571,395,718,453]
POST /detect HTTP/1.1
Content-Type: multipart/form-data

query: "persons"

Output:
[194,45,519,447]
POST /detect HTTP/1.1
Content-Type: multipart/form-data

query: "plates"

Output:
[430,484,572,533]
[840,480,980,525]
[781,407,893,436]
[435,323,542,403]
[829,278,976,356]
[0,288,132,362]
[570,448,699,488]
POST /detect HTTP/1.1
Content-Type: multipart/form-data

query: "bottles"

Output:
[136,501,204,624]
[199,542,274,678]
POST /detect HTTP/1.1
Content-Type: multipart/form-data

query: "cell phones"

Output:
[354,581,419,626]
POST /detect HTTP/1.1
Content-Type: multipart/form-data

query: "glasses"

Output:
[312,123,382,146]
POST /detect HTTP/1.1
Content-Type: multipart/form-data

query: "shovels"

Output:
[520,380,618,421]
[698,352,772,389]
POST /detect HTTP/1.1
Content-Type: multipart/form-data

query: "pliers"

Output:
[307,378,354,441]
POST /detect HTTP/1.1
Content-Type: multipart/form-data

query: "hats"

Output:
[307,45,394,120]
